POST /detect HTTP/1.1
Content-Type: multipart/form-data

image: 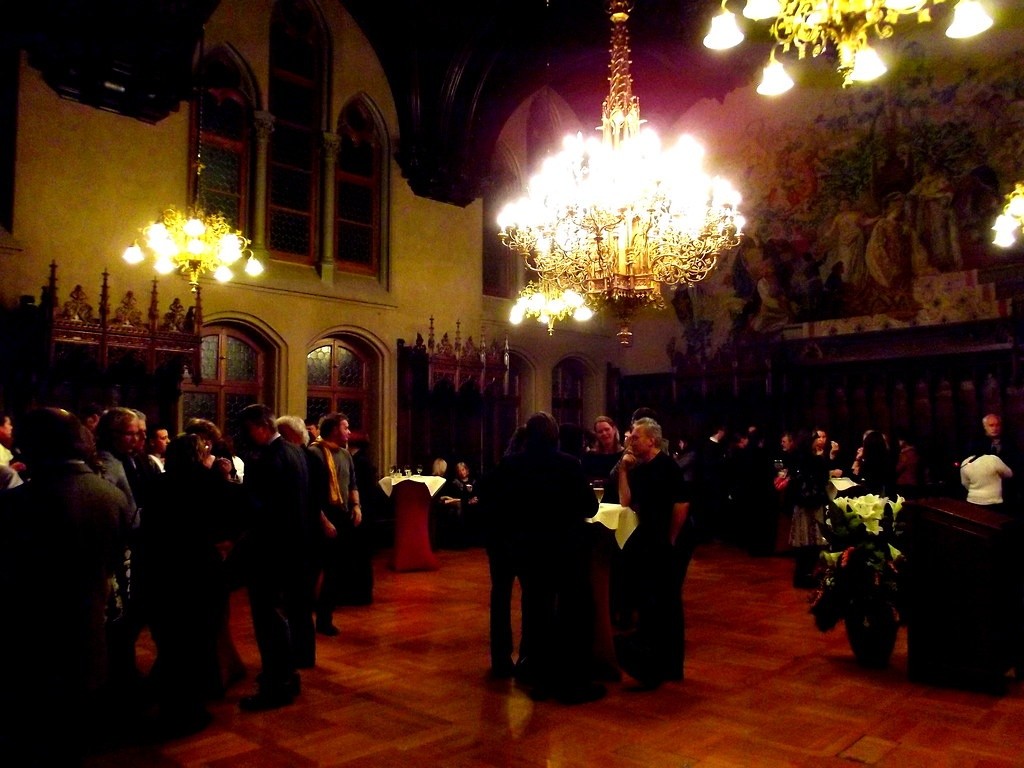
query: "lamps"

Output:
[496,0,1024,348]
[122,26,264,292]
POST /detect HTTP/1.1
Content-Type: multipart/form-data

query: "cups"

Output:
[404,465,411,475]
[396,464,403,473]
[389,466,394,478]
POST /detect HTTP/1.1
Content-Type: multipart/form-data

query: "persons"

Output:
[604,407,692,692]
[476,425,532,680]
[695,423,917,589]
[585,416,626,476]
[0,399,376,768]
[982,414,1019,503]
[959,435,1014,515]
[502,412,607,706]
[428,460,483,547]
[670,434,696,480]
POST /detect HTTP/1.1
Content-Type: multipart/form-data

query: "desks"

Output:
[583,503,639,665]
[378,475,447,571]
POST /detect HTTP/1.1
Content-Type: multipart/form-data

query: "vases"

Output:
[845,596,897,667]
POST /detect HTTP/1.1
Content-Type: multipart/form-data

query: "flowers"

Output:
[811,493,906,633]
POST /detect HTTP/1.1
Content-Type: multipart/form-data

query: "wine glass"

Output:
[593,480,605,509]
[416,464,423,475]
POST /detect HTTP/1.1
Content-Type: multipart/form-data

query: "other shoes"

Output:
[154,704,214,742]
[487,655,516,680]
[794,572,822,587]
[317,614,338,636]
[239,677,302,711]
